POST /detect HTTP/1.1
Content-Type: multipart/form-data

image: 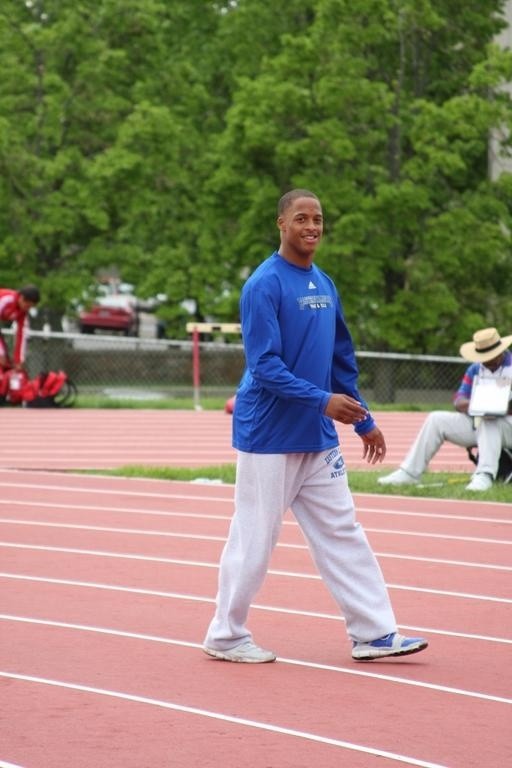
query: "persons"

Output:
[376,326,511,497]
[202,184,428,665]
[0,283,41,401]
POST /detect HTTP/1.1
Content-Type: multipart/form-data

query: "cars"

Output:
[76,293,140,337]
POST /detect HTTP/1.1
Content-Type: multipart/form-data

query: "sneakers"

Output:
[201,644,276,662]
[378,468,419,486]
[465,473,493,491]
[352,631,428,659]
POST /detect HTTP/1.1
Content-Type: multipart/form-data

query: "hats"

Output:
[460,326,511,363]
